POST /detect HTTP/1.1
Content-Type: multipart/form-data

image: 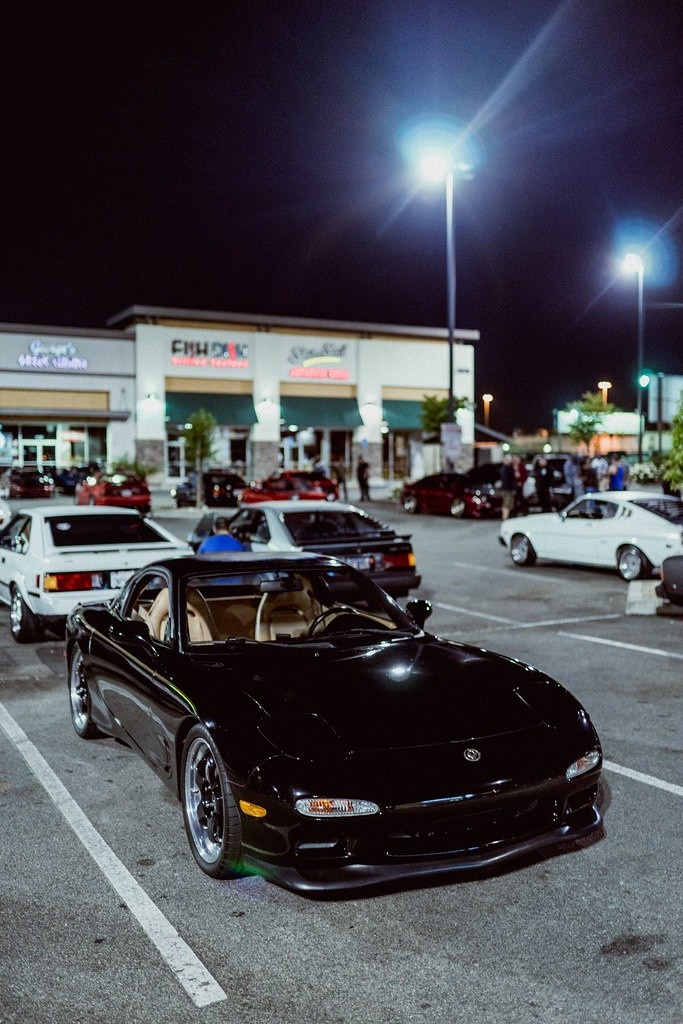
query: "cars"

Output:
[0,504,198,643]
[498,490,683,582]
[210,499,422,613]
[237,468,340,505]
[463,452,580,510]
[399,472,505,520]
[0,465,54,501]
[74,469,152,514]
[53,466,98,495]
[186,507,243,554]
[170,469,251,509]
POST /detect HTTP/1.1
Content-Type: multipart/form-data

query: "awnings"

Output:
[382,401,427,431]
[279,395,364,428]
[166,392,258,425]
[474,423,519,445]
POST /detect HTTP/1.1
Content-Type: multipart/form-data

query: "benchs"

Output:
[142,593,262,642]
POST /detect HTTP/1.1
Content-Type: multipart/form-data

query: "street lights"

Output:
[424,150,456,414]
[622,251,644,462]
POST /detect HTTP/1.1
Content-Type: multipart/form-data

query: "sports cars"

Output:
[65,550,605,892]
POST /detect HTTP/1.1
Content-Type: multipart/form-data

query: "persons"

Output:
[333,459,347,500]
[533,458,555,513]
[67,467,84,478]
[564,451,628,511]
[313,458,324,474]
[195,517,250,584]
[357,457,372,501]
[500,452,529,519]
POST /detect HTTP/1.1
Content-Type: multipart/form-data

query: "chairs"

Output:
[256,577,326,643]
[143,582,225,643]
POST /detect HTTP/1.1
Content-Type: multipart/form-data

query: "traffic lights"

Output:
[638,373,651,388]
